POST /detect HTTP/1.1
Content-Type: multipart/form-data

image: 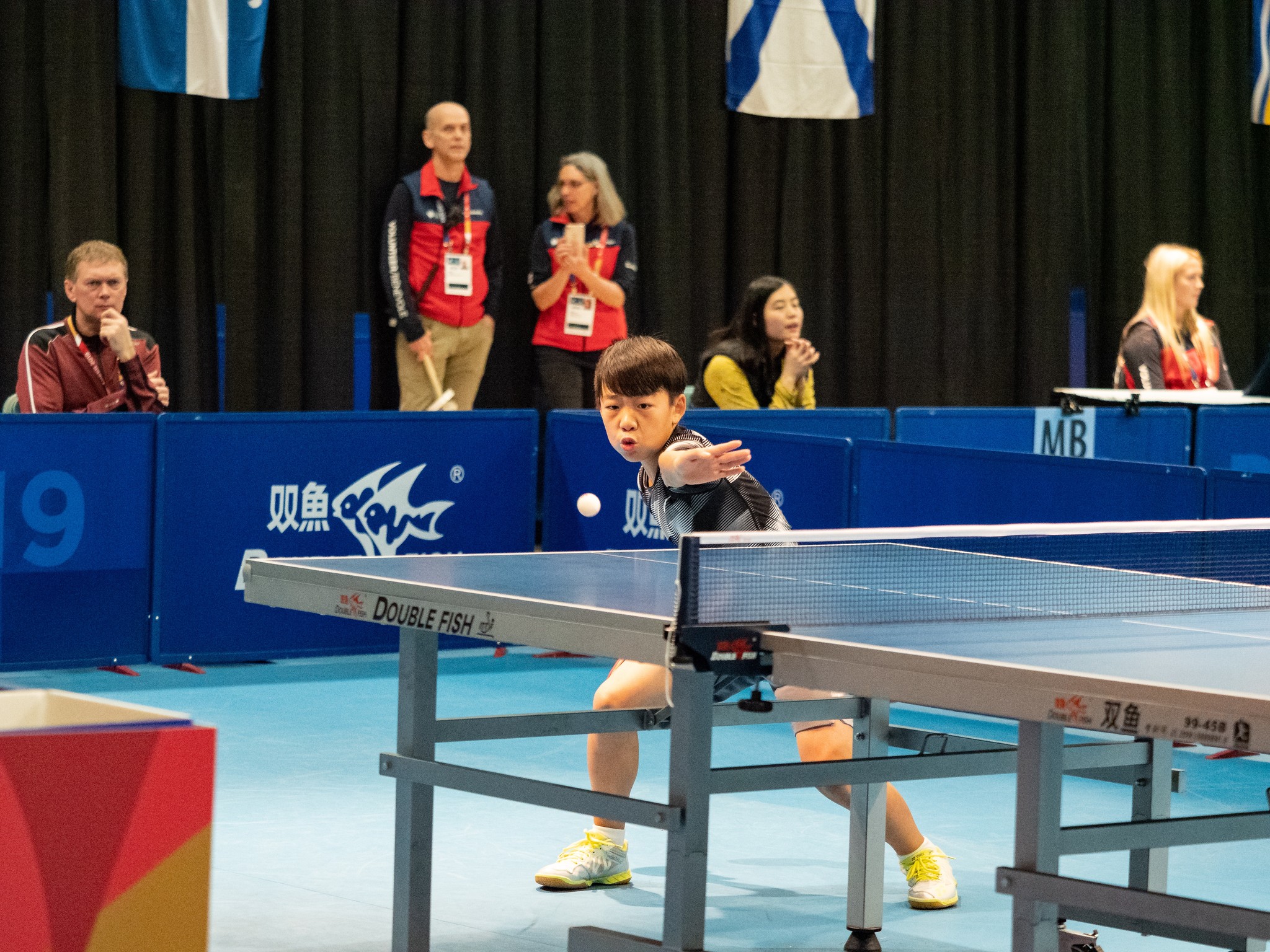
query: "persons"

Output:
[1114,243,1235,392]
[688,276,821,410]
[528,152,639,410]
[534,334,961,910]
[381,101,506,413]
[16,238,170,415]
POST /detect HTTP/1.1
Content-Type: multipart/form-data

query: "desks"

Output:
[242,539,1270,952]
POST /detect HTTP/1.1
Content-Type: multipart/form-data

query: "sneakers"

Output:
[535,831,632,889]
[899,844,958,909]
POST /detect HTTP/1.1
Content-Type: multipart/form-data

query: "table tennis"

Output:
[577,492,602,517]
